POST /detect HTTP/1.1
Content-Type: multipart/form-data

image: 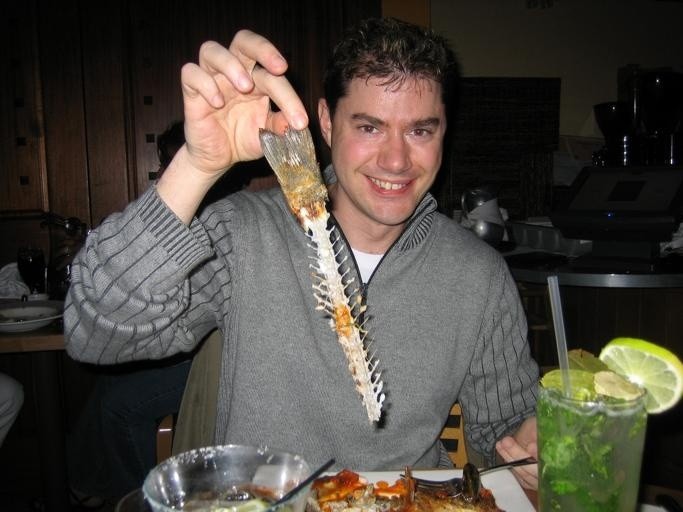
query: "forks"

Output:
[399,456,539,496]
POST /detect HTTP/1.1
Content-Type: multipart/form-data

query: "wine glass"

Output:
[17,247,45,293]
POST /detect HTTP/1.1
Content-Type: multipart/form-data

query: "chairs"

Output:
[437,405,470,471]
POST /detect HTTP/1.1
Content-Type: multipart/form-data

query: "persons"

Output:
[0,374,25,446]
[79,119,257,491]
[64,16,542,493]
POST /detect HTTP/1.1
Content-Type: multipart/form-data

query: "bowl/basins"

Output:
[142,442,312,512]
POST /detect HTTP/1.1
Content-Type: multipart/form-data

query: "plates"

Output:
[0,302,65,332]
[253,464,538,512]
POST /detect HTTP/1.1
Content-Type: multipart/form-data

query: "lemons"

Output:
[599,337,681,413]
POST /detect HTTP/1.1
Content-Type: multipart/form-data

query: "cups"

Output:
[28,293,48,302]
[534,379,647,512]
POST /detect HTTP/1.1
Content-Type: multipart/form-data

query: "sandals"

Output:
[26,479,105,508]
